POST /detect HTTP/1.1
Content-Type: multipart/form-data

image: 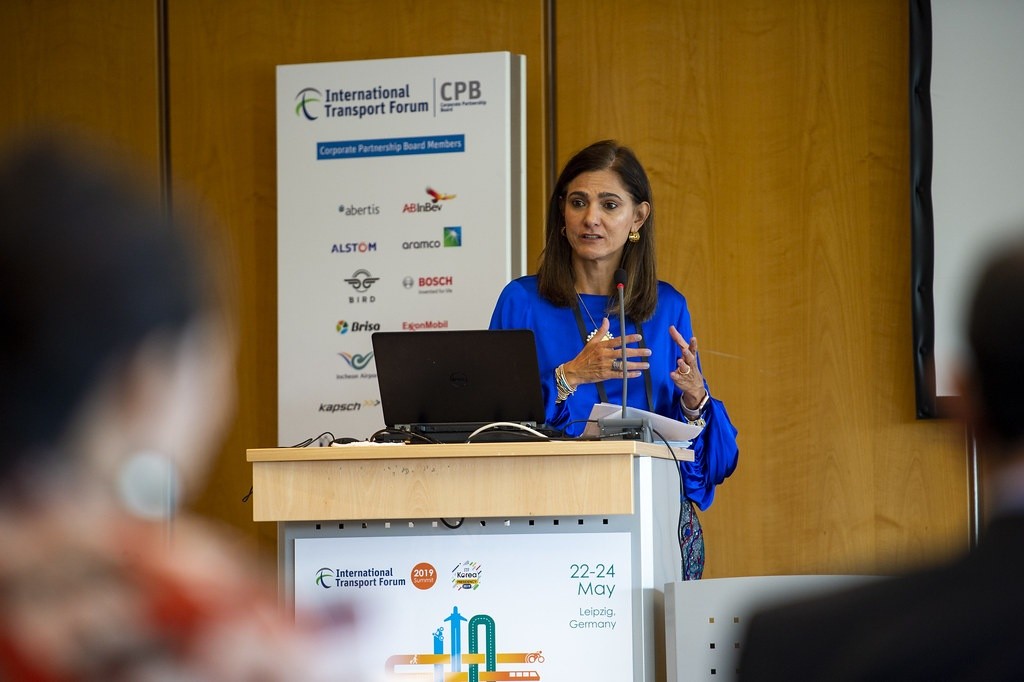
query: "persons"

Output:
[0,145,348,682]
[735,242,1024,682]
[489,137,741,580]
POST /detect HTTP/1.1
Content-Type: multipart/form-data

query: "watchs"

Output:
[681,388,709,416]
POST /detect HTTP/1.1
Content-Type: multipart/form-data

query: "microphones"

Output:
[597,268,654,444]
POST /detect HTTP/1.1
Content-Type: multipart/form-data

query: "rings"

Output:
[612,361,622,371]
[678,366,690,375]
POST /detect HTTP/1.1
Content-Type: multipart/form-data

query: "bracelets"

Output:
[554,364,576,404]
[684,416,706,426]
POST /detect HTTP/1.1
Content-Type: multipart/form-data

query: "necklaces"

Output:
[575,288,615,350]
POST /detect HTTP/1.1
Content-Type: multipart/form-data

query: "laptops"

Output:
[371,328,556,435]
